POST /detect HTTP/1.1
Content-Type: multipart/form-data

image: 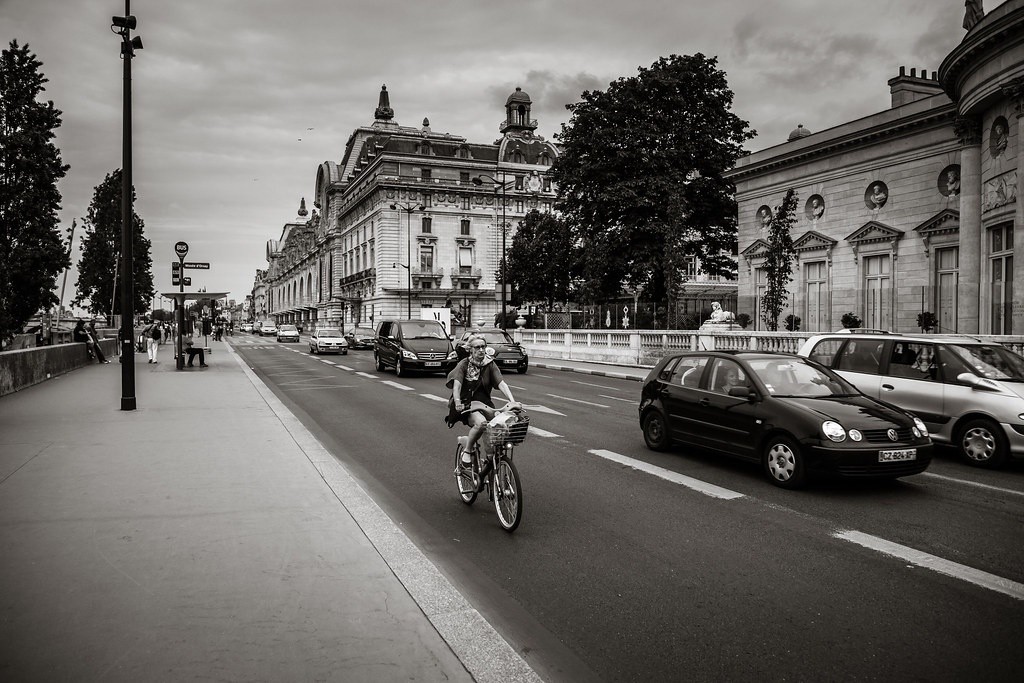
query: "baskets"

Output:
[479,416,530,450]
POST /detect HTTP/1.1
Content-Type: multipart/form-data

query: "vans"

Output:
[373,319,459,379]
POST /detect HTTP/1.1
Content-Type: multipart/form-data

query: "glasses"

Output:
[470,344,488,350]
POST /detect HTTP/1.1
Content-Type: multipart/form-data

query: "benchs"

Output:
[175,352,186,367]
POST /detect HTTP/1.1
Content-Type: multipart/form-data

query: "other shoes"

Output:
[99,360,111,364]
[187,362,194,367]
[199,363,208,367]
[487,486,502,501]
[460,450,473,470]
[148,359,158,364]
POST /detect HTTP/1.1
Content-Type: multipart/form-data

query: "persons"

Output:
[86,320,111,364]
[118,319,224,368]
[446,333,523,500]
[962,0,985,31]
[710,302,725,321]
[74,319,95,358]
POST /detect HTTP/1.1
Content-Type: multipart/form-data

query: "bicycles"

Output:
[454,401,529,532]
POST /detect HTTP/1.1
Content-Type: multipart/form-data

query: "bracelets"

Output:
[454,398,461,402]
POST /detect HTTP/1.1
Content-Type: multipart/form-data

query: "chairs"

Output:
[846,354,863,370]
[932,350,947,364]
[673,365,766,395]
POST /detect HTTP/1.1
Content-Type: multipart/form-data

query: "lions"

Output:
[705,302,735,323]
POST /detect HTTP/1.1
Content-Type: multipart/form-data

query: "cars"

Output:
[251,322,261,334]
[234,325,239,331]
[309,329,348,355]
[277,325,299,342]
[244,324,253,332]
[455,329,529,374]
[135,315,149,324]
[638,350,933,487]
[343,327,376,350]
[240,324,246,332]
[91,315,107,323]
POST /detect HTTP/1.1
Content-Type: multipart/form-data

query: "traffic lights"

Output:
[341,302,344,310]
[369,316,373,320]
[339,317,343,320]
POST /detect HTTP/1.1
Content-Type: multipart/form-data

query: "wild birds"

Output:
[296,139,302,142]
[307,128,314,131]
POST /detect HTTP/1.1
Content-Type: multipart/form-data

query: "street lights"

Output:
[390,174,515,332]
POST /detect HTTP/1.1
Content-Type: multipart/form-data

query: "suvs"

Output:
[259,321,278,337]
[789,328,1024,467]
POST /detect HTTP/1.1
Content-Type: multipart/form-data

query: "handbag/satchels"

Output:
[445,384,474,429]
[152,326,161,339]
